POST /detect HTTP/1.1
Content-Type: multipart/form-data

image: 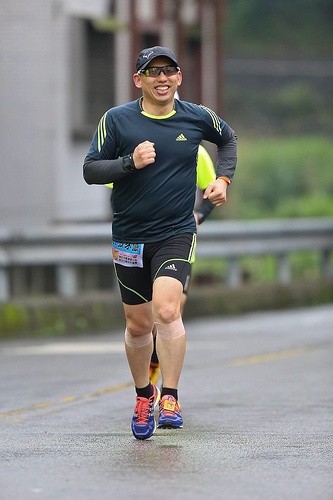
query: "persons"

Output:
[83,46,237,439]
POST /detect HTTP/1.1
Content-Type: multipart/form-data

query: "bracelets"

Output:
[217,176,231,186]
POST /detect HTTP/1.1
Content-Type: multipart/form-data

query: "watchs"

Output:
[122,153,135,170]
